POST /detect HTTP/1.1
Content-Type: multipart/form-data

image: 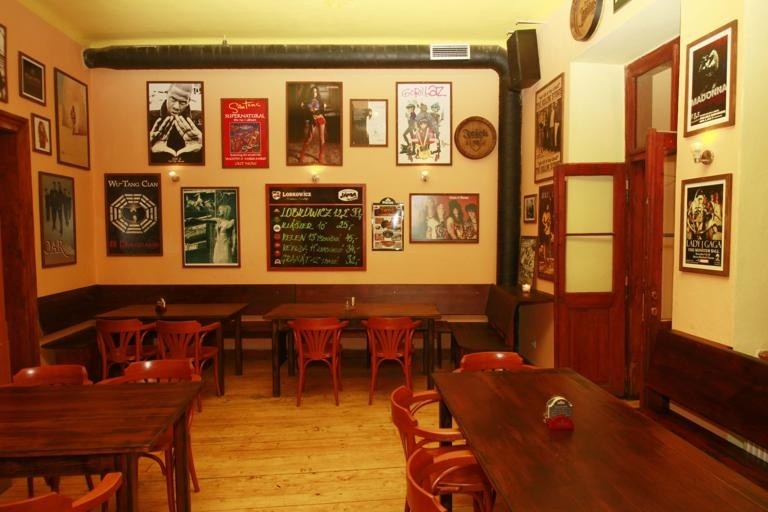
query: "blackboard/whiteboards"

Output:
[266,183,366,271]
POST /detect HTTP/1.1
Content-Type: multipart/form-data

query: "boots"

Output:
[317,143,326,163]
[299,143,308,161]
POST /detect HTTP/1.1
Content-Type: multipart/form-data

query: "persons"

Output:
[299,85,327,164]
[398,101,446,161]
[415,195,477,239]
[525,198,534,217]
[547,103,555,151]
[183,205,235,263]
[70,105,76,136]
[541,202,552,258]
[44,181,72,236]
[148,82,203,163]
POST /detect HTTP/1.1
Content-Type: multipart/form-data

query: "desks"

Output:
[243,300,553,396]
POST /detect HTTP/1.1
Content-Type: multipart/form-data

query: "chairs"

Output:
[0,295,247,512]
[289,318,349,407]
[361,316,421,405]
[391,316,767,510]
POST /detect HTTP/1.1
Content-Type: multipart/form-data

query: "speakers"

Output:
[506,29,540,88]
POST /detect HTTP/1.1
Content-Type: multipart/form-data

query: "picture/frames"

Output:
[0,22,10,107]
[395,81,452,166]
[350,99,388,147]
[454,116,497,160]
[29,111,53,157]
[514,73,566,298]
[372,197,405,252]
[146,80,206,167]
[219,97,269,169]
[103,172,163,257]
[409,192,480,244]
[682,18,738,138]
[679,171,733,278]
[53,63,91,172]
[179,186,242,269]
[16,49,47,108]
[285,81,344,167]
[36,167,79,270]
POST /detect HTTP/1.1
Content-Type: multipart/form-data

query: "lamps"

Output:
[687,142,717,165]
